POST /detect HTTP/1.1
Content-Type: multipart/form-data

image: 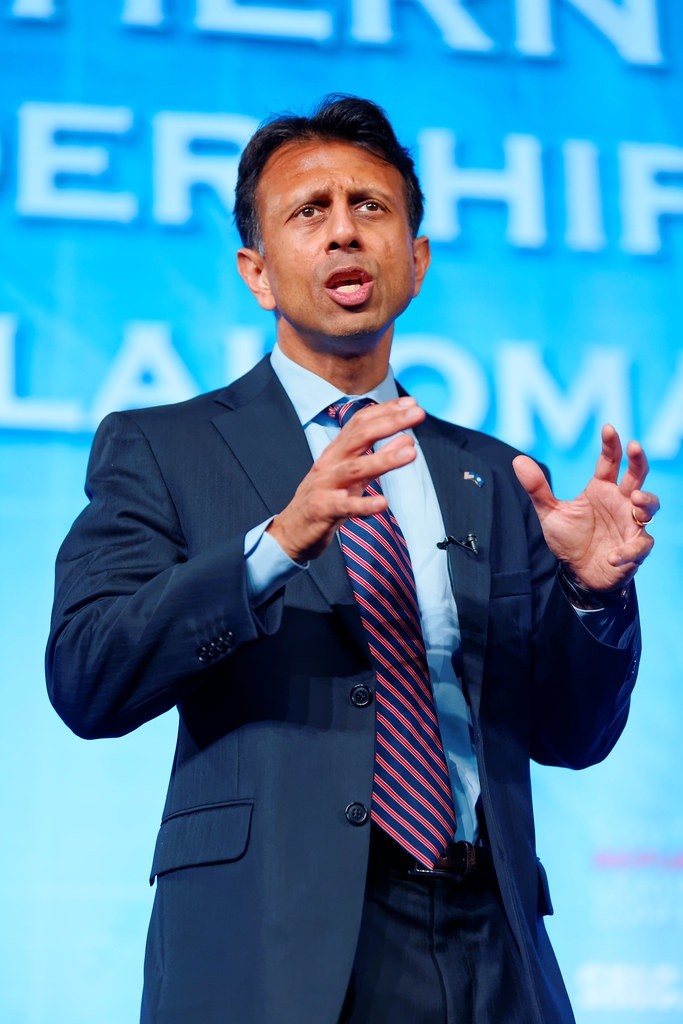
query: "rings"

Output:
[632,509,654,526]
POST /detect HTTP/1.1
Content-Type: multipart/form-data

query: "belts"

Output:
[367,840,497,876]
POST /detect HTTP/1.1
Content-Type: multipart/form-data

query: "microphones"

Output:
[465,531,479,556]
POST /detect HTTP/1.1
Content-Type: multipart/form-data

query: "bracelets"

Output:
[558,563,629,607]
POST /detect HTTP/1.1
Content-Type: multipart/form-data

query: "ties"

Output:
[327,398,457,870]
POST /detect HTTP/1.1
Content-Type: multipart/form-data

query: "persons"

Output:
[45,95,660,1023]
